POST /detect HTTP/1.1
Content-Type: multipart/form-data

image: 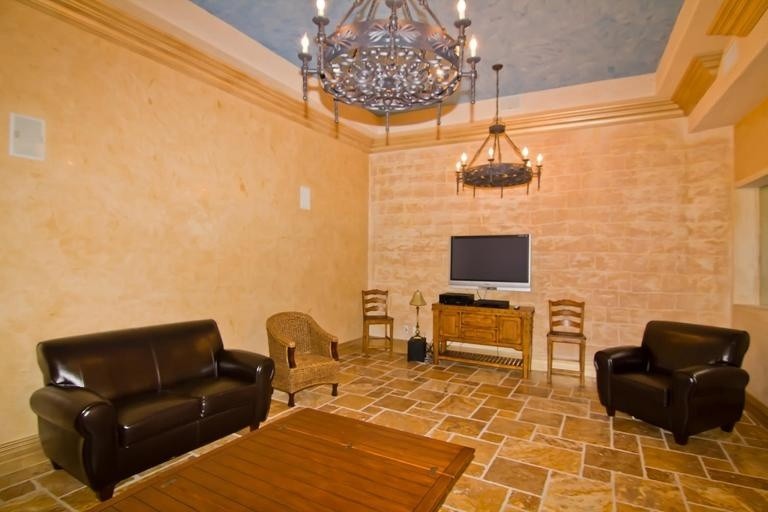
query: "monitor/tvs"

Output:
[449,234,531,292]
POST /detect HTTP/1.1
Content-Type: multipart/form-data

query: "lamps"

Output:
[406,287,426,340]
[298,1,481,126]
[455,62,544,199]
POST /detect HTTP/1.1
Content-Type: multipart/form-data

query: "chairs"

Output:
[592,318,751,446]
[359,287,395,356]
[262,308,339,408]
[545,298,588,389]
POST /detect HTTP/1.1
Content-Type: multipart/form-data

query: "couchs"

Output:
[28,316,275,503]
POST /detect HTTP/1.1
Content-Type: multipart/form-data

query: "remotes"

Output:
[514,304,520,310]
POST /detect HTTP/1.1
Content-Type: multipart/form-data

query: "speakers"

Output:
[408,337,426,361]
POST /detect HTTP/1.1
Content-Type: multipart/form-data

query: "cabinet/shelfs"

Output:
[406,336,427,363]
[430,300,536,378]
